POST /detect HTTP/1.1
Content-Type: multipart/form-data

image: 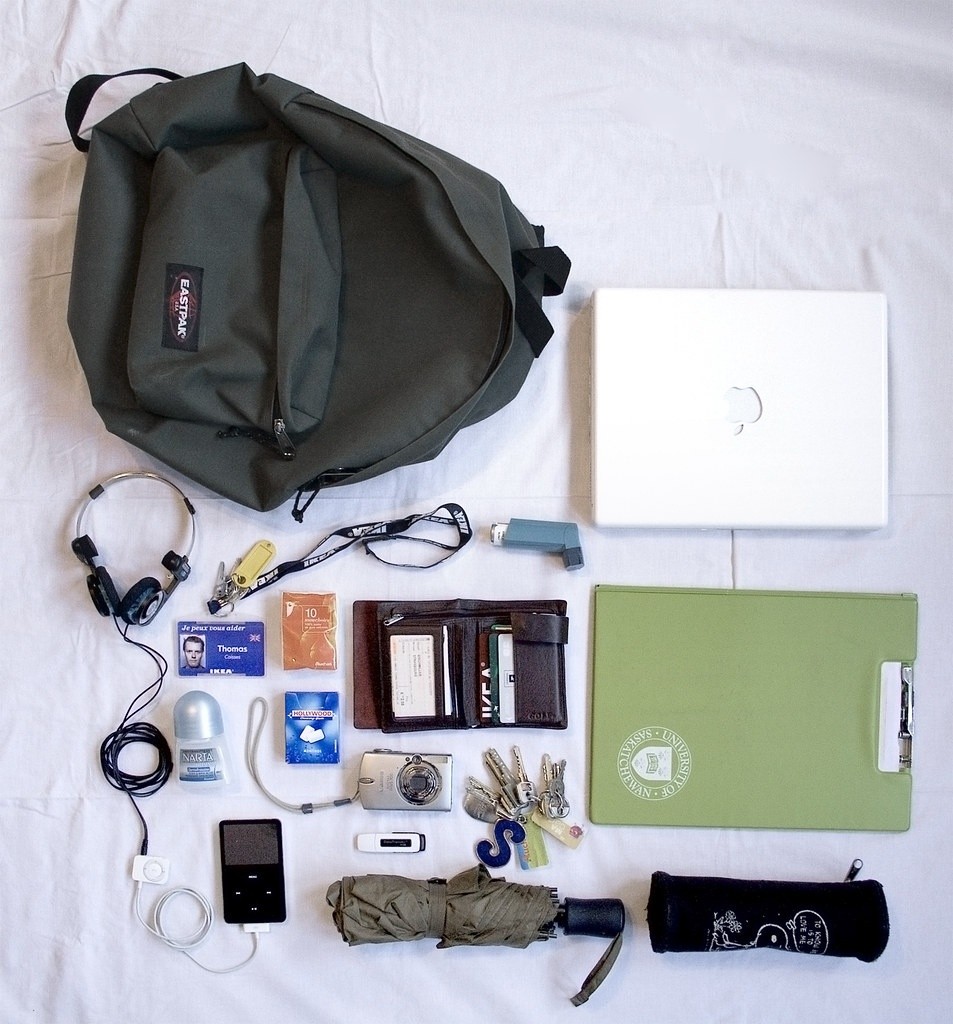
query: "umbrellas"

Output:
[325,863,625,1006]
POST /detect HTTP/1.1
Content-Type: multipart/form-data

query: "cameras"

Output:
[358,748,454,812]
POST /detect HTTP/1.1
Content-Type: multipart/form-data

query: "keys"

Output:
[465,747,567,823]
[213,557,248,602]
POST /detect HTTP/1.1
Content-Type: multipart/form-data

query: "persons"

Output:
[181,636,206,670]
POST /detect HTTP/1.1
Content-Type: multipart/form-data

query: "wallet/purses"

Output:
[353,598,568,734]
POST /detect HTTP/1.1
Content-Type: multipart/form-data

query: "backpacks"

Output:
[64,61,572,522]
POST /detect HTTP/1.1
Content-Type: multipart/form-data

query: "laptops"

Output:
[589,287,888,531]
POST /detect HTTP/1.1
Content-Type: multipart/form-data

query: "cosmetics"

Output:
[173,690,233,794]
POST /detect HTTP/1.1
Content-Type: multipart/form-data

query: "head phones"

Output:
[73,471,196,626]
